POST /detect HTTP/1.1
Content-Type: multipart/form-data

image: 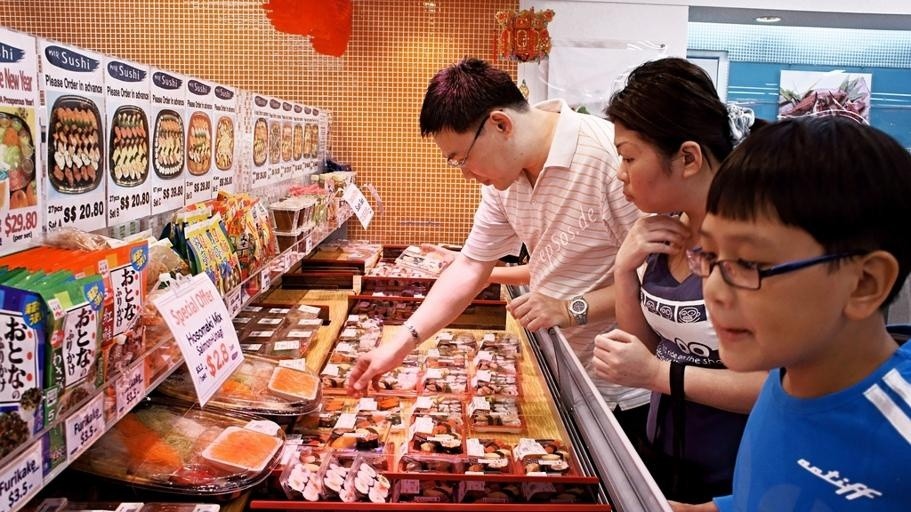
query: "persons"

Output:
[591,57,773,502]
[668,111,910,511]
[345,58,656,451]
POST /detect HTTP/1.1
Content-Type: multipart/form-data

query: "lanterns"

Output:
[493,8,558,99]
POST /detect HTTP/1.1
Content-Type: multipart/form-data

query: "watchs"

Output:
[568,293,592,325]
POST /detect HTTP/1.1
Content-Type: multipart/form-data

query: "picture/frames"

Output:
[685,49,729,106]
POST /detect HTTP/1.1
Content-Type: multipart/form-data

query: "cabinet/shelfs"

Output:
[0,204,672,512]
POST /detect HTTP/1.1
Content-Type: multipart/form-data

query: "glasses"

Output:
[685,245,871,290]
[447,108,504,168]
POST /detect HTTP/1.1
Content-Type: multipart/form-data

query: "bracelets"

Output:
[667,357,690,402]
[401,318,422,350]
